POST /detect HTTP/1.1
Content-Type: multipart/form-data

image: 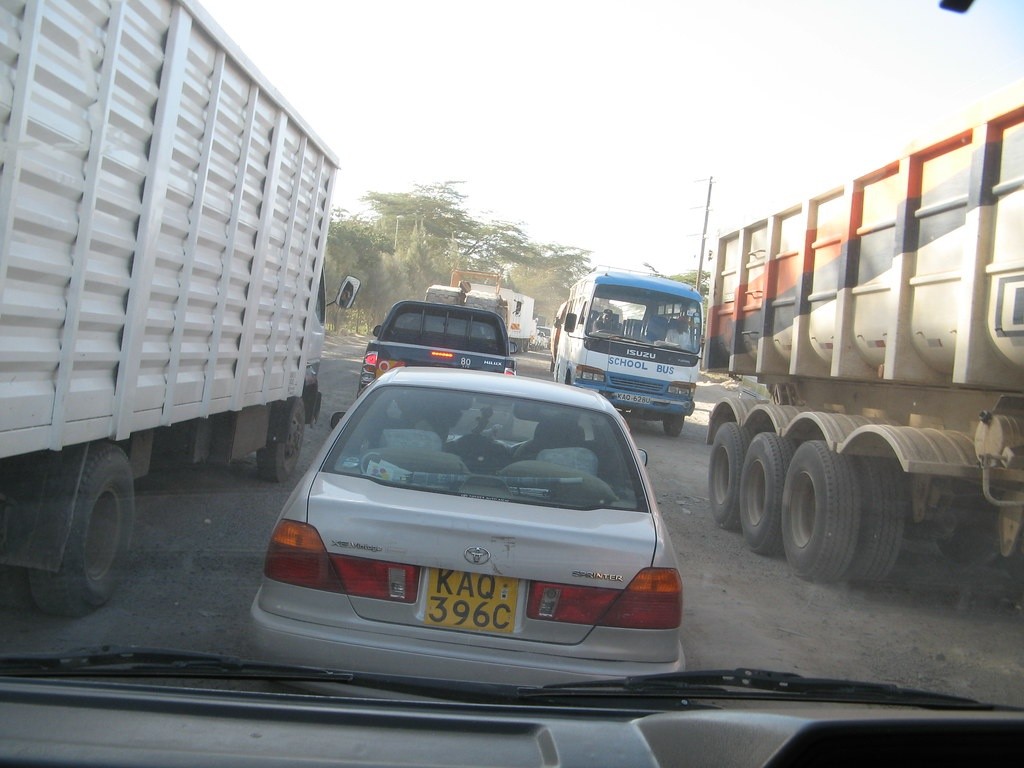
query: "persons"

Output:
[593,309,617,331]
[665,316,691,348]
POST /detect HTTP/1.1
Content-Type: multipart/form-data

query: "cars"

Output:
[249,366,689,695]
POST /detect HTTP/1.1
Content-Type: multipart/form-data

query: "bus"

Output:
[550,264,703,437]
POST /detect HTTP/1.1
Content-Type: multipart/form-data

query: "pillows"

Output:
[359,447,471,492]
[495,459,619,507]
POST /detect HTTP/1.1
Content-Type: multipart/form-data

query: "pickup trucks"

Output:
[357,300,516,399]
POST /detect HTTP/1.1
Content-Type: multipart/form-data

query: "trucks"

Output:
[425,268,534,352]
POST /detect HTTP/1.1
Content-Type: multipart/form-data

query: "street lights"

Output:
[395,214,403,249]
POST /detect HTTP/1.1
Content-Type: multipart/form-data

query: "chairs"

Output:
[536,448,598,476]
[667,318,678,331]
[586,309,669,343]
[379,429,442,455]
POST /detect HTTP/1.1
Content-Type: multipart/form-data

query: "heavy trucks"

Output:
[0,0,360,619]
[699,85,1024,590]
